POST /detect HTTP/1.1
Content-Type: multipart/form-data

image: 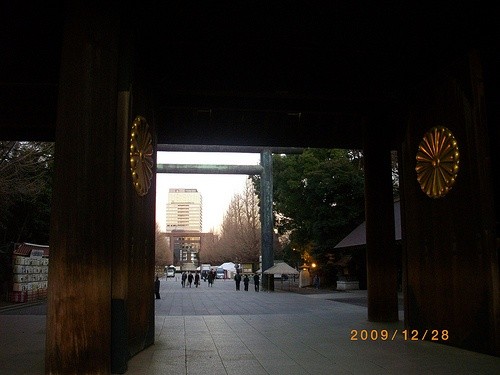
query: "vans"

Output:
[216,268,225,279]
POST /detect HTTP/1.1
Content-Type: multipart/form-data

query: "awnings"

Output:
[334,203,402,248]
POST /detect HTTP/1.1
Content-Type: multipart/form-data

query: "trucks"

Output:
[200,264,212,280]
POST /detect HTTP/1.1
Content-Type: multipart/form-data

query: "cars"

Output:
[167,269,175,277]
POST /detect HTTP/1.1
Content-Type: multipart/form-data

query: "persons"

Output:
[208,269,216,287]
[254,273,259,292]
[181,271,187,288]
[195,273,199,288]
[188,273,194,288]
[154,277,160,299]
[243,275,249,291]
[234,271,241,290]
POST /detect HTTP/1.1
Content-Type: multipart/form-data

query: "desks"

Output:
[337,281,360,290]
